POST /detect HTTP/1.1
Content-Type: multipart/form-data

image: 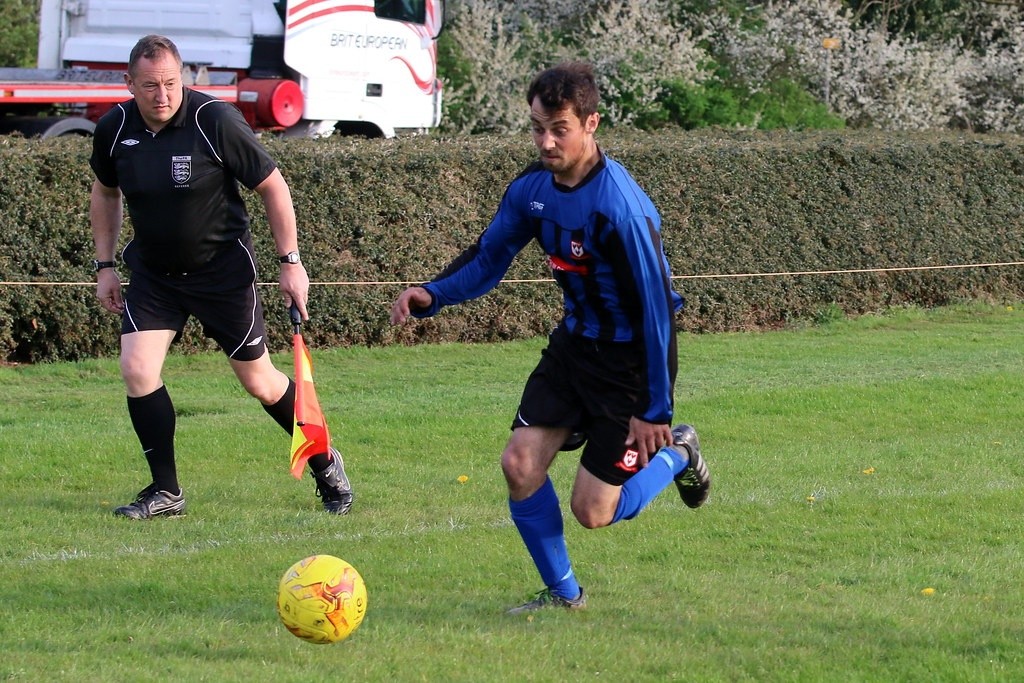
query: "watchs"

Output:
[93,259,117,273]
[279,251,301,265]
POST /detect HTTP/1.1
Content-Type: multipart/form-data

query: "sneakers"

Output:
[671,423,711,508]
[506,586,587,613]
[115,482,187,519]
[309,446,354,516]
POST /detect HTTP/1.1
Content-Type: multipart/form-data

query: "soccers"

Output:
[275,553,370,647]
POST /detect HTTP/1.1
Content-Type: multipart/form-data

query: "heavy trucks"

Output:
[2,0,447,143]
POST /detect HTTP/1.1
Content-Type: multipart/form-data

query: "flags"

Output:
[289,332,332,481]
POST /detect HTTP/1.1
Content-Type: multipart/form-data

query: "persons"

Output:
[88,34,355,520]
[388,58,712,618]
